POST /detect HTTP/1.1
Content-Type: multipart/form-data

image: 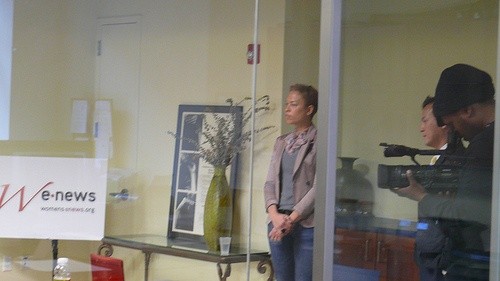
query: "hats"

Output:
[432,62,496,128]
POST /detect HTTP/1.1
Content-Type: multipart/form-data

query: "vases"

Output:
[335,156,360,219]
[203,165,233,254]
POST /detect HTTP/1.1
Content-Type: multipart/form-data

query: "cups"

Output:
[219,237,231,257]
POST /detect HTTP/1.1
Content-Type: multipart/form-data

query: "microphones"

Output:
[383,146,407,157]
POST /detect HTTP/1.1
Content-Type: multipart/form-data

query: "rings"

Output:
[281,229,285,232]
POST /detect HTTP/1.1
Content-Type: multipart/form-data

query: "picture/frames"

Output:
[166,104,243,245]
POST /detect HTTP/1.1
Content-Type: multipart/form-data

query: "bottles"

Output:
[53,258,72,281]
[336,156,363,210]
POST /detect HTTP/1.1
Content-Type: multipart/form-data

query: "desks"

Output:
[97,232,274,281]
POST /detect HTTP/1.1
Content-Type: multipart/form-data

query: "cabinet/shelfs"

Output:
[334,227,417,281]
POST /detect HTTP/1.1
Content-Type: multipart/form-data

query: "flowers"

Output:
[167,95,275,166]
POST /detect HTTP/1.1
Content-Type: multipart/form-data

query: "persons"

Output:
[390,64,495,281]
[264,83,326,281]
